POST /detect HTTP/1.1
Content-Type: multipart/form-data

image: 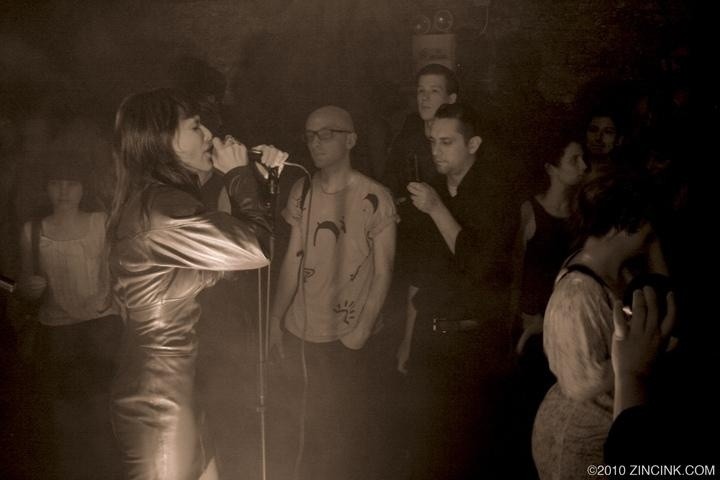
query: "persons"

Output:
[0,65,720,480]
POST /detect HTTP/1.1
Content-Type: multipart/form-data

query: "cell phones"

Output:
[406,151,421,183]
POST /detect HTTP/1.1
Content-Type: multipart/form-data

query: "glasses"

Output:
[302,128,354,142]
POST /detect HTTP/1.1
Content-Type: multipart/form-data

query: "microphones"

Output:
[212,147,263,161]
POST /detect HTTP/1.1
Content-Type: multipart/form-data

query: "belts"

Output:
[417,316,485,335]
